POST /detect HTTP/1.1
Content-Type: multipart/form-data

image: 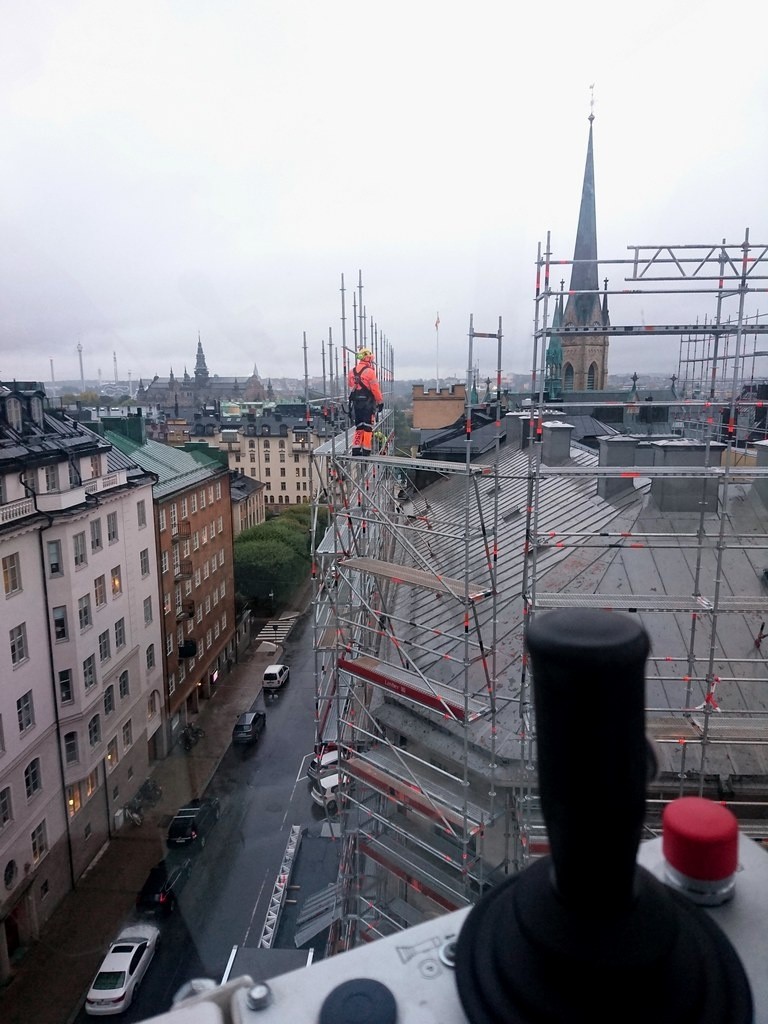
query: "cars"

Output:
[83,922,162,1016]
[135,851,192,915]
[320,554,342,590]
[163,794,219,852]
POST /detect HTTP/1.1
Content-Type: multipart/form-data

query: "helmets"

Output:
[356,348,371,362]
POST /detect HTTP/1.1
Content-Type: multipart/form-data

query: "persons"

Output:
[348,348,384,456]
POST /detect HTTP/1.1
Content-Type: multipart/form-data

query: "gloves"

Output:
[376,403,383,412]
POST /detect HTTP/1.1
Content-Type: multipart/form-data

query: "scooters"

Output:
[138,778,162,808]
[175,720,207,755]
[119,796,146,828]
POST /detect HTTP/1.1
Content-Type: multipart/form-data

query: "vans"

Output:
[261,664,290,691]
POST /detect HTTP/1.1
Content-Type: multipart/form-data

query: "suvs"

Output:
[312,770,353,816]
[231,711,266,746]
[307,749,346,784]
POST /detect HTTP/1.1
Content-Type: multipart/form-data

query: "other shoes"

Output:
[363,449,371,456]
[352,447,362,456]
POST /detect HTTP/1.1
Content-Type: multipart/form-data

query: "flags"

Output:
[435,316,440,331]
